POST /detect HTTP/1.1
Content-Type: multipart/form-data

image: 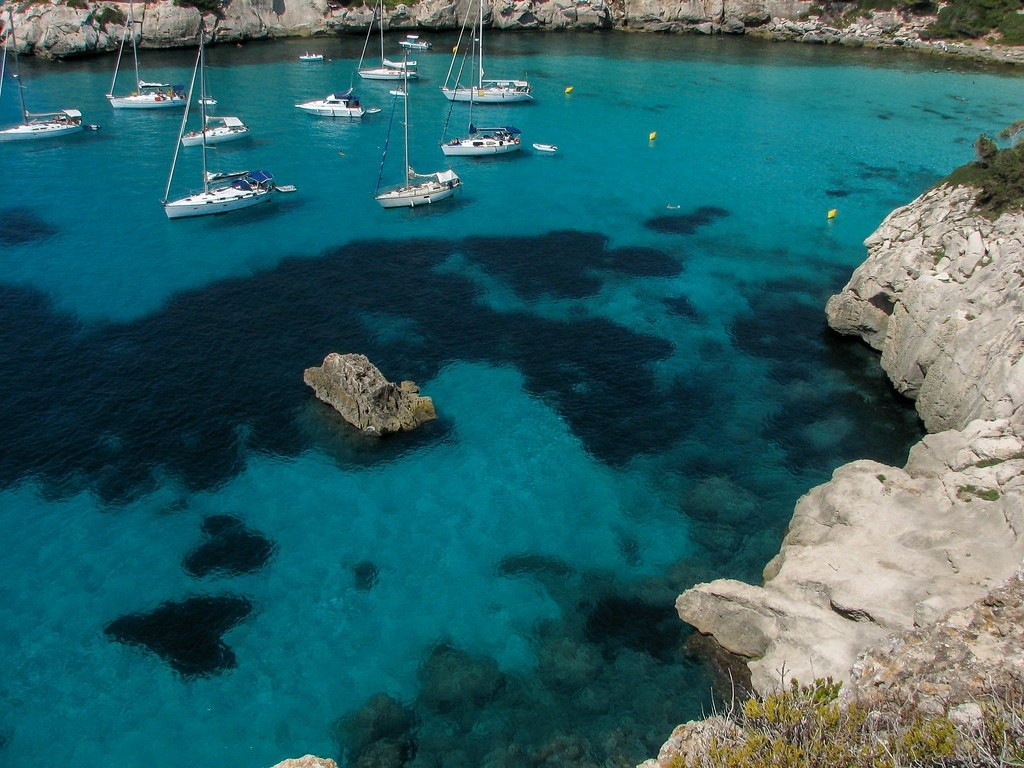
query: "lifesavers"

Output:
[514,138,520,145]
[516,87,520,91]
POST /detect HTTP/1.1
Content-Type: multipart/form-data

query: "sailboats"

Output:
[158,30,281,221]
[372,44,464,208]
[104,0,188,109]
[356,0,418,80]
[439,24,522,156]
[438,0,534,104]
[0,6,85,142]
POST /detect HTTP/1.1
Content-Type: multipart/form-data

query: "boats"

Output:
[180,114,250,147]
[383,58,417,71]
[295,87,366,118]
[298,51,326,62]
[199,97,217,105]
[532,143,558,152]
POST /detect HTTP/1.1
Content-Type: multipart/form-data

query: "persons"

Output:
[495,132,514,142]
[53,114,78,125]
[249,179,265,191]
[155,87,178,100]
[451,137,463,146]
[190,124,209,137]
[446,178,460,189]
